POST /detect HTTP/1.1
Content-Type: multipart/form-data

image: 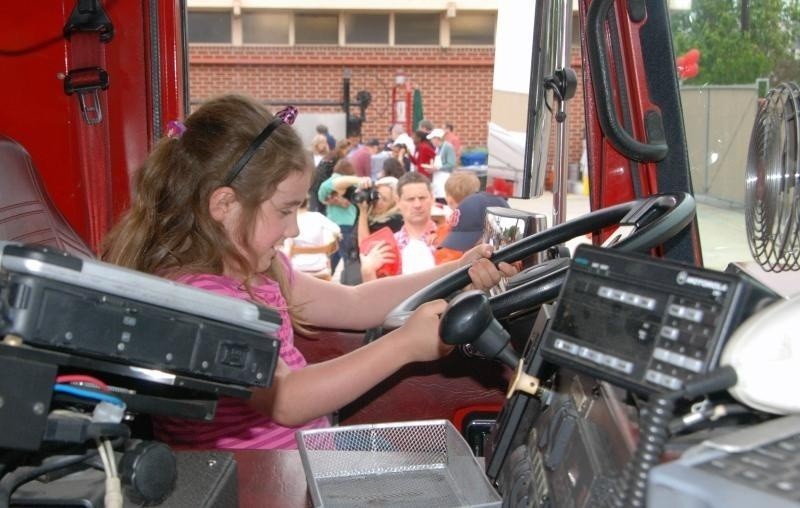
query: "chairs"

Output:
[0,134,94,263]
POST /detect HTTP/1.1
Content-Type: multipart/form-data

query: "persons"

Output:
[97,94,523,448]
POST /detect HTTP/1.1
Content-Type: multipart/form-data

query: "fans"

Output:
[741,80,800,274]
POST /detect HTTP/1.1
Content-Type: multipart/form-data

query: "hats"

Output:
[425,128,444,139]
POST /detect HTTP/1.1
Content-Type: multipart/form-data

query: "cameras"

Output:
[349,189,378,203]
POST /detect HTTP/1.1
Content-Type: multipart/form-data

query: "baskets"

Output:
[296,419,504,507]
